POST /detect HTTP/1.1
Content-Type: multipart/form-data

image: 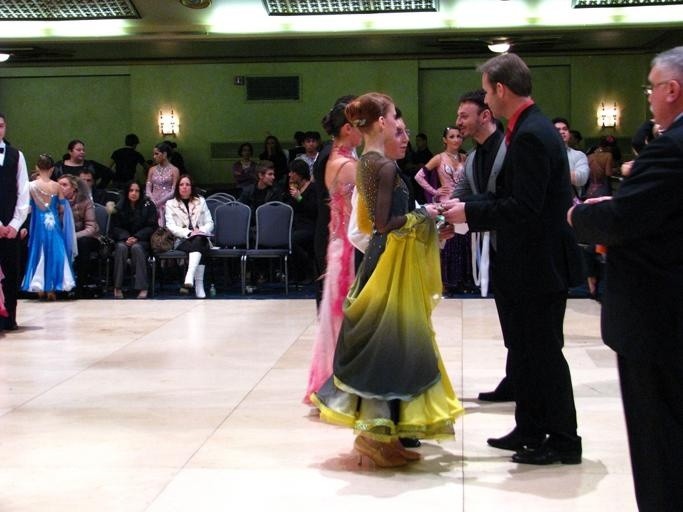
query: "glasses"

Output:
[643,81,668,95]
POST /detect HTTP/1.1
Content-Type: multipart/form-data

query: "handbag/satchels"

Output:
[150,227,174,252]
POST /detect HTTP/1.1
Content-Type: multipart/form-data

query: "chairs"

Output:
[94,188,296,299]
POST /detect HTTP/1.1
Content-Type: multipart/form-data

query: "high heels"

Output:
[38,291,55,301]
[355,435,420,467]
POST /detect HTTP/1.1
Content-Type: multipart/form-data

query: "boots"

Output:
[184,252,206,298]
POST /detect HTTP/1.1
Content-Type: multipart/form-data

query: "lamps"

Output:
[157,105,182,140]
[487,37,515,54]
[596,98,622,133]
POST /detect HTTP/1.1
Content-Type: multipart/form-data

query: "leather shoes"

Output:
[487,427,581,465]
[137,290,148,298]
[114,287,123,299]
[479,384,514,402]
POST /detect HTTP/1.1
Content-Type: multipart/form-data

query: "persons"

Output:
[345,107,455,258]
[308,91,468,469]
[433,92,516,402]
[564,45,681,512]
[229,131,335,231]
[0,116,32,331]
[438,54,581,463]
[553,118,663,297]
[400,125,475,299]
[20,134,214,302]
[304,94,364,406]
